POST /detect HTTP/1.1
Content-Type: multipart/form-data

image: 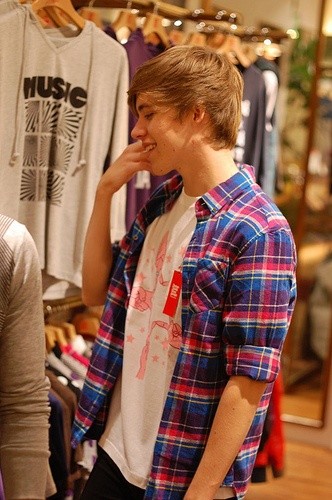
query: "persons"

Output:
[66,46,300,498]
[2,210,59,499]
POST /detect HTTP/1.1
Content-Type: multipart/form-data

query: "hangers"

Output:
[43,310,109,412]
[0,0,284,80]
